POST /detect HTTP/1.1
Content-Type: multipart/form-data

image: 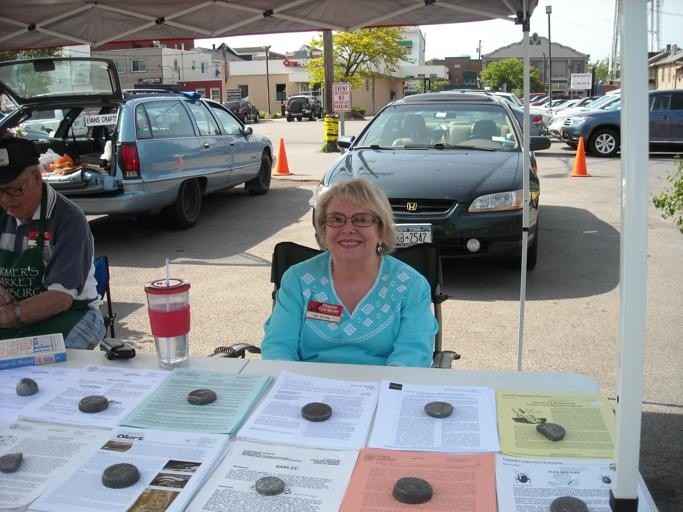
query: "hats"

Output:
[0,136,40,185]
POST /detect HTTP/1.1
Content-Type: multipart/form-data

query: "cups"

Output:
[146,279,191,366]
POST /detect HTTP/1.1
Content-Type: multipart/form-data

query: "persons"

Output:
[0,137,107,350]
[261,178,440,368]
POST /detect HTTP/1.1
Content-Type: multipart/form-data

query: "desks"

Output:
[0,350,658,512]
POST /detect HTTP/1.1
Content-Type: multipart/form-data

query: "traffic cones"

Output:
[570,137,591,176]
[274,139,294,177]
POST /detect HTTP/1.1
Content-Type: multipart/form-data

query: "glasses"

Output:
[322,212,381,227]
[0,170,35,198]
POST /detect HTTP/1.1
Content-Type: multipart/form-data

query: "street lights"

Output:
[265,46,270,114]
[546,5,552,104]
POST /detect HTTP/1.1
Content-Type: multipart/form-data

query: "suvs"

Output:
[223,101,259,123]
[287,95,323,122]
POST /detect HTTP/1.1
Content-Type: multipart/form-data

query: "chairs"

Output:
[93,256,115,338]
[391,115,502,149]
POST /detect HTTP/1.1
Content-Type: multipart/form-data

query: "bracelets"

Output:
[13,301,20,327]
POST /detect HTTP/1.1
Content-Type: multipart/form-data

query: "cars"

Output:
[520,90,683,156]
[0,56,276,231]
